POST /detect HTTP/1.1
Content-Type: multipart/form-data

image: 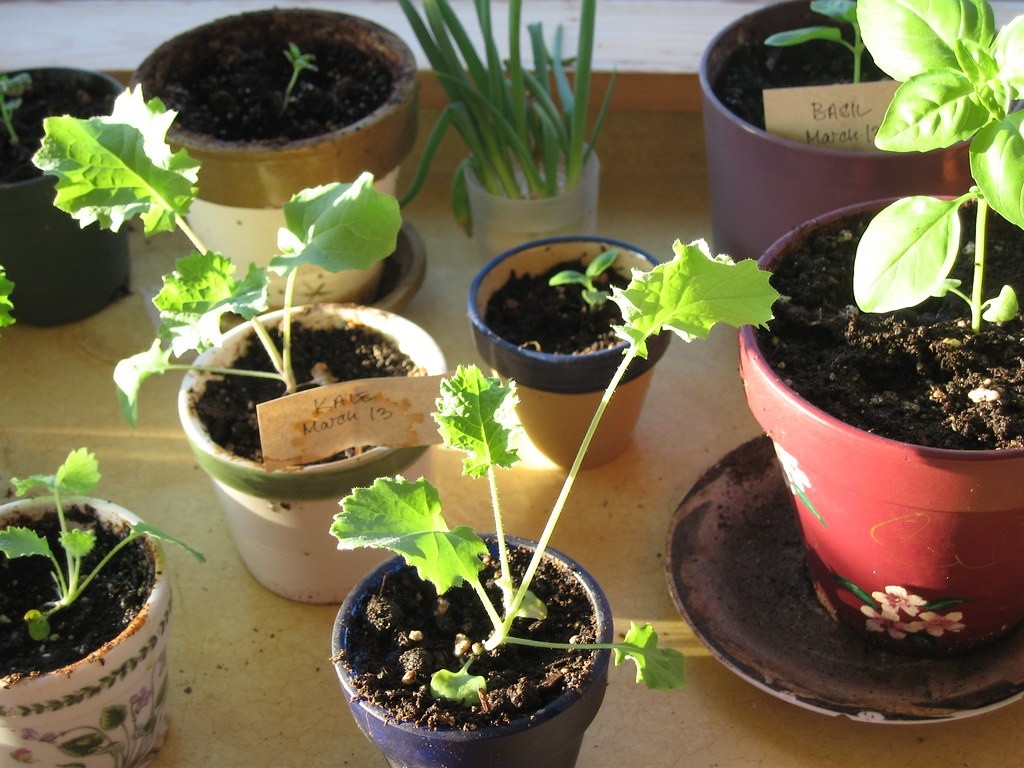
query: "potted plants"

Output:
[0,59,133,332]
[392,0,606,263]
[26,78,452,613]
[328,236,783,768]
[128,13,432,315]
[701,0,976,266]
[737,0,1024,652]
[0,446,207,768]
[463,232,668,481]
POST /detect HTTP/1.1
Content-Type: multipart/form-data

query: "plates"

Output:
[666,433,1023,725]
